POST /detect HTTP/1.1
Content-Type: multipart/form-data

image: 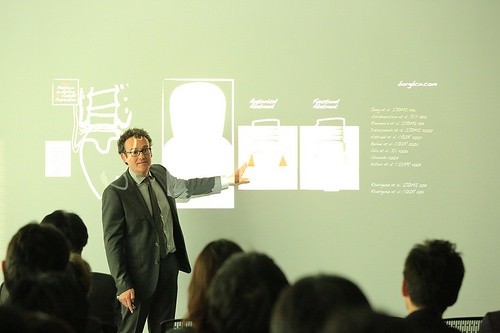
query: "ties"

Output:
[144,177,168,255]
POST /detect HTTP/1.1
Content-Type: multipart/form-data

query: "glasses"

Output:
[122,148,152,157]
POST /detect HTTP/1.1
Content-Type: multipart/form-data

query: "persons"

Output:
[0,210,120,333]
[102,128,251,333]
[169,239,500,333]
